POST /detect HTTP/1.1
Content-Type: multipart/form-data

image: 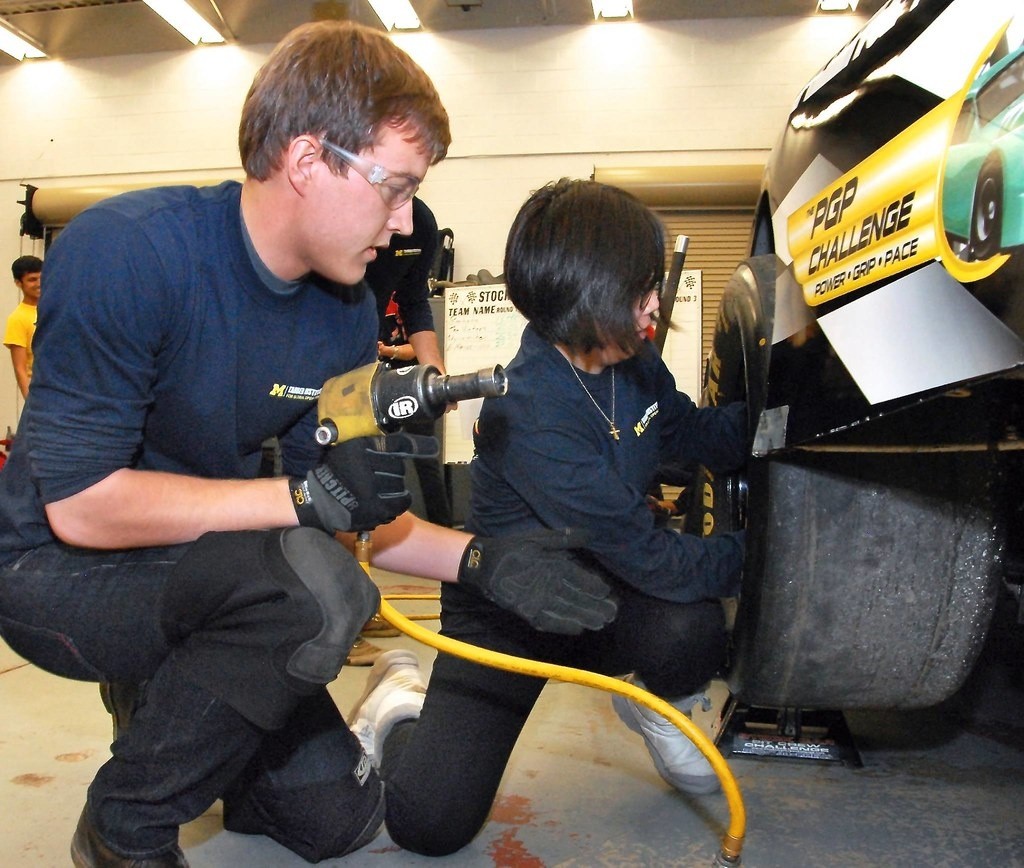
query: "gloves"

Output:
[458,530,619,632]
[288,432,440,538]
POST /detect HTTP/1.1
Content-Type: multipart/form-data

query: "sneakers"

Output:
[612,672,721,794]
[346,649,427,776]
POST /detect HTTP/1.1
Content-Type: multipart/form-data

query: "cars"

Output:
[709,1,1023,719]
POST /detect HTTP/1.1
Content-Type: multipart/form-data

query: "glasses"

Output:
[639,277,664,310]
[316,139,420,210]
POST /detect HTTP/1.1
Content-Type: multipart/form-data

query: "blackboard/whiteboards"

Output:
[442,267,705,467]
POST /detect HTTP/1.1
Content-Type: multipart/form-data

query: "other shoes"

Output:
[99,680,131,744]
[346,635,388,665]
[358,614,402,638]
[71,800,188,868]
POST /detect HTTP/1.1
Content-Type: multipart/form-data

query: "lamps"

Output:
[142,0,228,45]
[0,16,50,62]
[367,0,425,32]
[591,0,634,20]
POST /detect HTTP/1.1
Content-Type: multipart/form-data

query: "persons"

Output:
[1,19,622,868]
[347,179,754,859]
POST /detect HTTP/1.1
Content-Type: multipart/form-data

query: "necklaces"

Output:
[556,345,622,442]
